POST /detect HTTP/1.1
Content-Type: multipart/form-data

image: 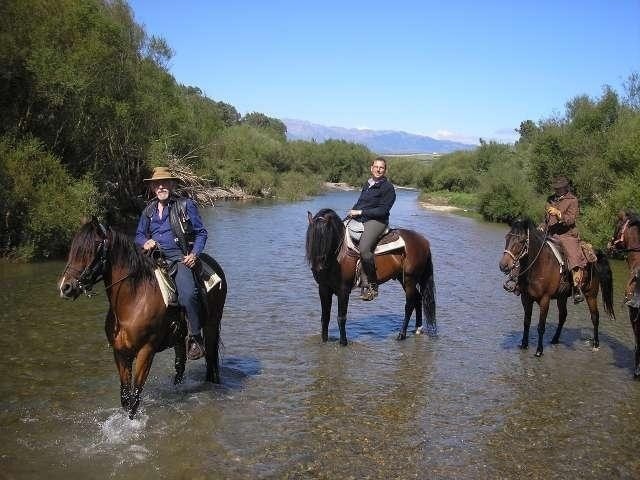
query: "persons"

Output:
[135,166,209,361]
[503,174,586,306]
[344,157,397,302]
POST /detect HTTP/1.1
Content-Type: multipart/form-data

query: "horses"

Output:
[499,214,616,357]
[606,210,640,380]
[57,214,227,422]
[303,208,436,349]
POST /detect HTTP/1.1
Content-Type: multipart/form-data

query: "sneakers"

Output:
[574,285,584,305]
[187,334,205,360]
[359,281,378,301]
[514,283,520,296]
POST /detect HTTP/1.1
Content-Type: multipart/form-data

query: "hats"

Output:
[143,167,178,183]
[551,174,570,189]
